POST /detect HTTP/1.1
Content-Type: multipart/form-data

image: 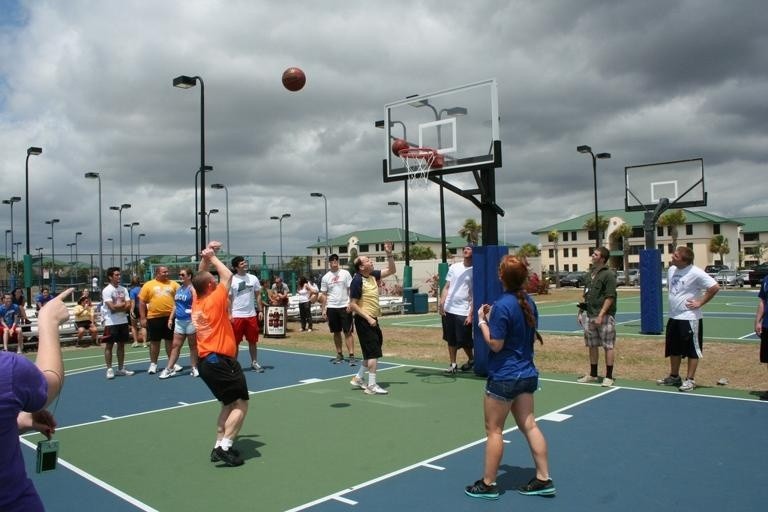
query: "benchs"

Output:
[0,303,104,348]
[285,294,412,322]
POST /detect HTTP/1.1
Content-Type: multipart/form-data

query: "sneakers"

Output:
[517,478,557,496]
[465,478,499,499]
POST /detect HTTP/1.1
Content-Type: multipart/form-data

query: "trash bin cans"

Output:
[401,287,429,314]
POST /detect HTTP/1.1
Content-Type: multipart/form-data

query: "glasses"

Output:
[179,274,185,277]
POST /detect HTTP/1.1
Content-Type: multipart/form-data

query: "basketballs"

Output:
[392,139,409,156]
[282,67,306,91]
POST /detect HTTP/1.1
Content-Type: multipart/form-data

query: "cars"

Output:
[543,262,767,288]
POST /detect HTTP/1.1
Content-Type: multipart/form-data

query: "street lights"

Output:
[0,196,23,293]
[84,172,105,292]
[74,231,82,282]
[107,238,115,268]
[387,201,405,260]
[45,218,60,294]
[270,213,292,271]
[24,146,43,309]
[576,144,612,249]
[66,242,77,284]
[211,183,230,266]
[170,77,219,272]
[35,247,44,290]
[123,222,140,270]
[109,203,131,272]
[137,233,146,268]
[404,93,469,263]
[373,119,410,266]
[310,192,329,273]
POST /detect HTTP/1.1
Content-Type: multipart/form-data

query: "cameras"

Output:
[36,439,59,473]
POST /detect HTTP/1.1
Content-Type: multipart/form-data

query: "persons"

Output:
[755,275,767,363]
[657,247,721,392]
[1,254,355,379]
[576,247,617,387]
[192,240,249,467]
[439,242,474,376]
[464,255,556,501]
[0,287,75,512]
[349,239,396,396]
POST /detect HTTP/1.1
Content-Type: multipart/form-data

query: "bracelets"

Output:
[477,321,487,329]
